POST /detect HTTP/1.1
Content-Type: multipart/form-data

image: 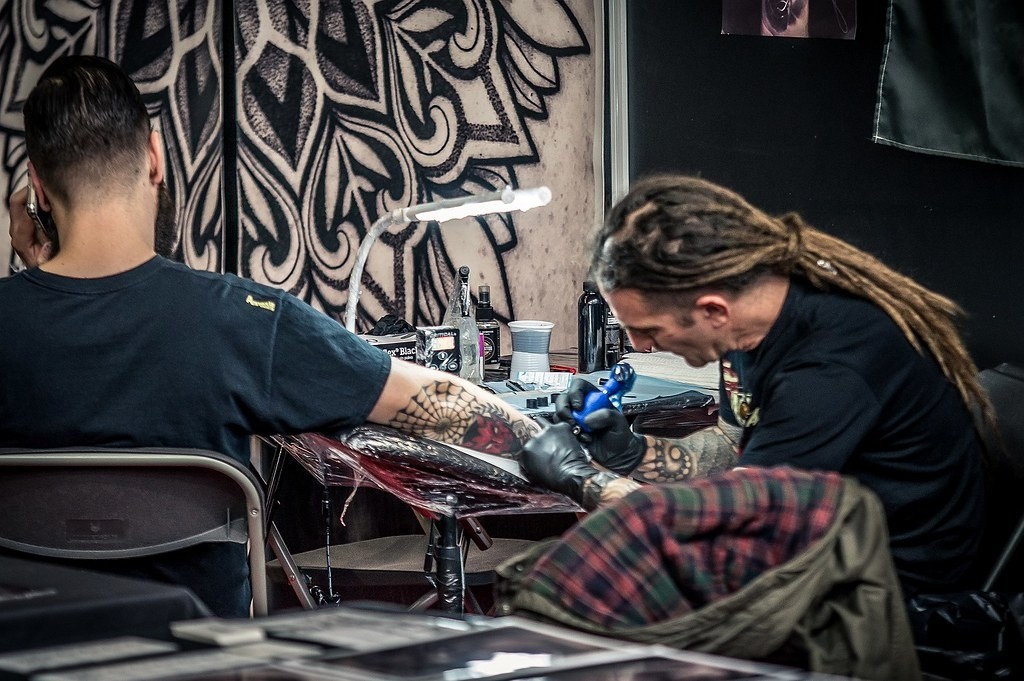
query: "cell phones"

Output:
[25,168,60,258]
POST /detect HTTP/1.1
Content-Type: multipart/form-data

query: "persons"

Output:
[0,54,549,460]
[516,174,1012,681]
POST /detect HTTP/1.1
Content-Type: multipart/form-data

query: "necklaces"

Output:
[734,348,760,418]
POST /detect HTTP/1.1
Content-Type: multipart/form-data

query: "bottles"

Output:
[476,287,501,369]
[452,264,481,381]
[577,281,606,374]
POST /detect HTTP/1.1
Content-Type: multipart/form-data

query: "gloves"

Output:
[515,420,596,501]
[552,377,647,476]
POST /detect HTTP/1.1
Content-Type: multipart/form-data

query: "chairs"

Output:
[1,444,270,629]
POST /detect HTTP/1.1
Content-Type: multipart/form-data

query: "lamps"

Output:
[342,186,551,335]
